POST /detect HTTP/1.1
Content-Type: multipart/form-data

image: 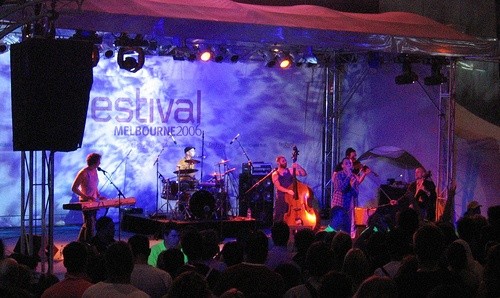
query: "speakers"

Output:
[10,37,94,152]
[14,234,58,261]
[239,173,274,228]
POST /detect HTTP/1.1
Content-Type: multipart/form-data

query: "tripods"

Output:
[151,166,193,220]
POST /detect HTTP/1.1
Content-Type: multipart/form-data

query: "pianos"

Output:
[63,197,136,243]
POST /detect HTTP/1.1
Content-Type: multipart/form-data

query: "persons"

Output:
[72,152,107,241]
[331,147,372,233]
[272,156,307,223]
[390,166,436,220]
[0,201,500,298]
[176,147,195,211]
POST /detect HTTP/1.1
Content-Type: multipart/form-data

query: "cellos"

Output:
[409,170,432,209]
[284,146,320,234]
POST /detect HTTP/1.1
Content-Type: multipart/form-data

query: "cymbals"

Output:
[173,169,198,173]
[185,160,200,163]
[209,174,224,176]
[214,160,230,165]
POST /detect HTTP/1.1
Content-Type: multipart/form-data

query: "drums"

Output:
[180,180,195,191]
[185,190,215,218]
[161,181,178,200]
[200,183,216,192]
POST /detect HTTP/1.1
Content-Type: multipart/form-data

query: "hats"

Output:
[344,247,370,272]
[468,201,482,209]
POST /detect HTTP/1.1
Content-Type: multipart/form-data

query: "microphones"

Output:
[169,132,177,144]
[98,167,106,172]
[230,134,240,144]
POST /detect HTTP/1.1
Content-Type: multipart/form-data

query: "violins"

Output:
[353,160,376,177]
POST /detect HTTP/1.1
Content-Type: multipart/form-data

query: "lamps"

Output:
[264,48,318,70]
[114,32,149,73]
[101,43,115,59]
[189,44,240,65]
[0,37,10,54]
[423,60,446,86]
[394,59,418,85]
